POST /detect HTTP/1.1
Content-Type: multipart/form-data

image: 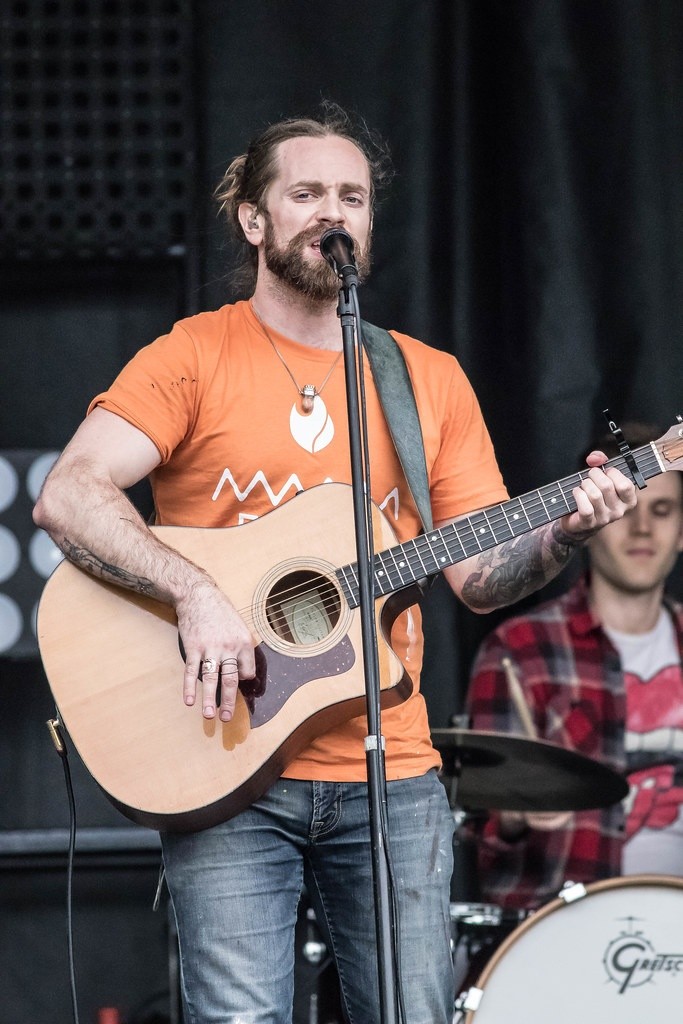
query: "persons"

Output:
[31,103,641,1024]
[448,420,683,919]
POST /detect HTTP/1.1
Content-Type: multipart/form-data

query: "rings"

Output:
[201,659,219,674]
[220,657,239,675]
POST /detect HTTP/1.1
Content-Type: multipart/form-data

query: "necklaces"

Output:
[250,300,343,412]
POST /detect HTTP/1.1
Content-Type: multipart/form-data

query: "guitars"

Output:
[33,409,682,835]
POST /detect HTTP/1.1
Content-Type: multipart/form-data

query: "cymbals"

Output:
[429,728,630,813]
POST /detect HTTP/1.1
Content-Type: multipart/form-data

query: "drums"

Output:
[449,870,681,1023]
[303,895,538,1023]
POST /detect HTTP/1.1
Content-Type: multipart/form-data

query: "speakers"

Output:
[0,827,182,1024]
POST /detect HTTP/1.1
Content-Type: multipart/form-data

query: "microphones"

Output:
[321,227,358,287]
